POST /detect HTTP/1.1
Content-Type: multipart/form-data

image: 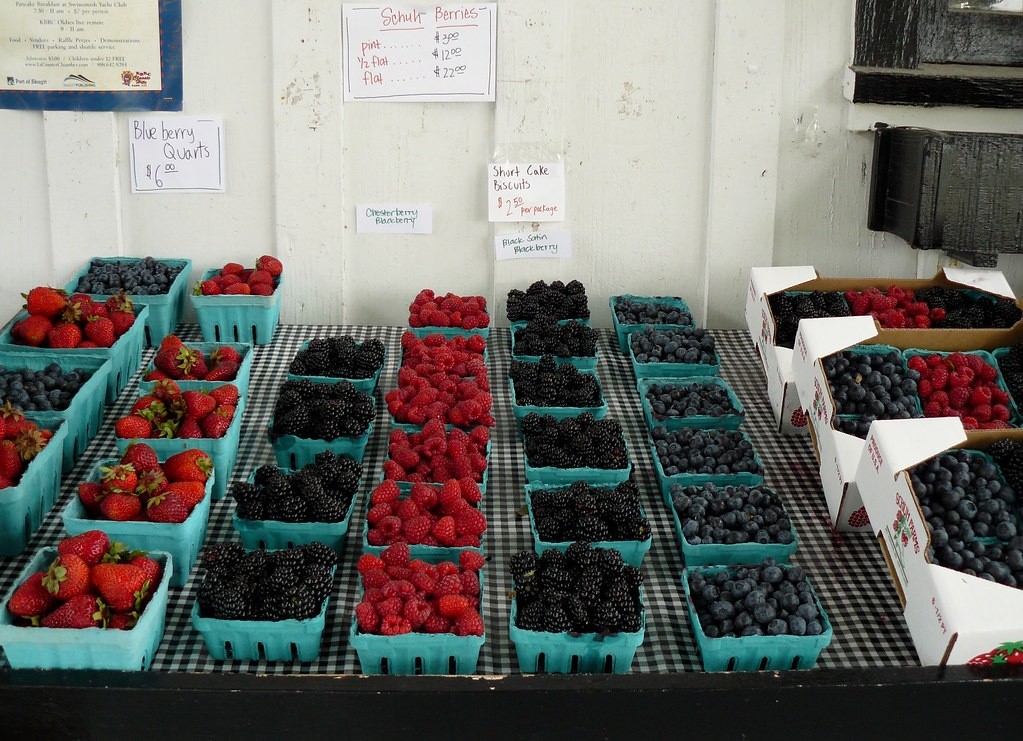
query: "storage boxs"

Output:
[0,253,1022,664]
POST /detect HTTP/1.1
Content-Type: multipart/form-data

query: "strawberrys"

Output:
[0,256,282,632]
